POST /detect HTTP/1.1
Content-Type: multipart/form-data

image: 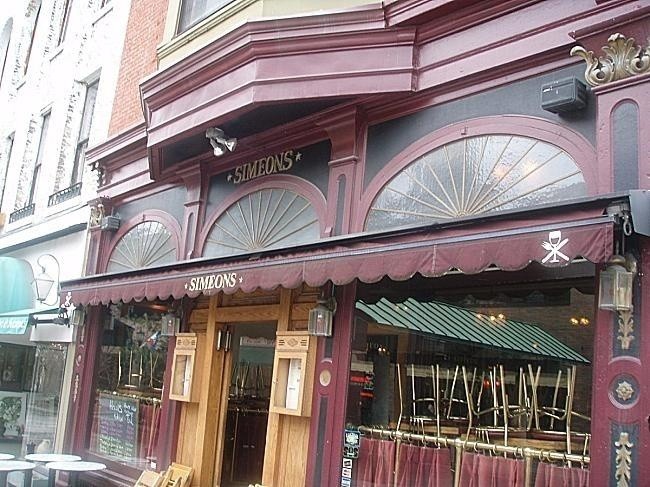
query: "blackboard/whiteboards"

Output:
[95,392,140,467]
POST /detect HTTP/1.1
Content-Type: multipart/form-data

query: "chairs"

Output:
[112,347,167,396]
[394,360,590,467]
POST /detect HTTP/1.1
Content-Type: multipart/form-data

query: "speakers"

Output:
[540,76,586,115]
[102,216,120,232]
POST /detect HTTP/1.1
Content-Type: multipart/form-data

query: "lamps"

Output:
[599,250,633,311]
[28,249,61,307]
[158,298,182,338]
[202,127,239,159]
[305,286,334,337]
